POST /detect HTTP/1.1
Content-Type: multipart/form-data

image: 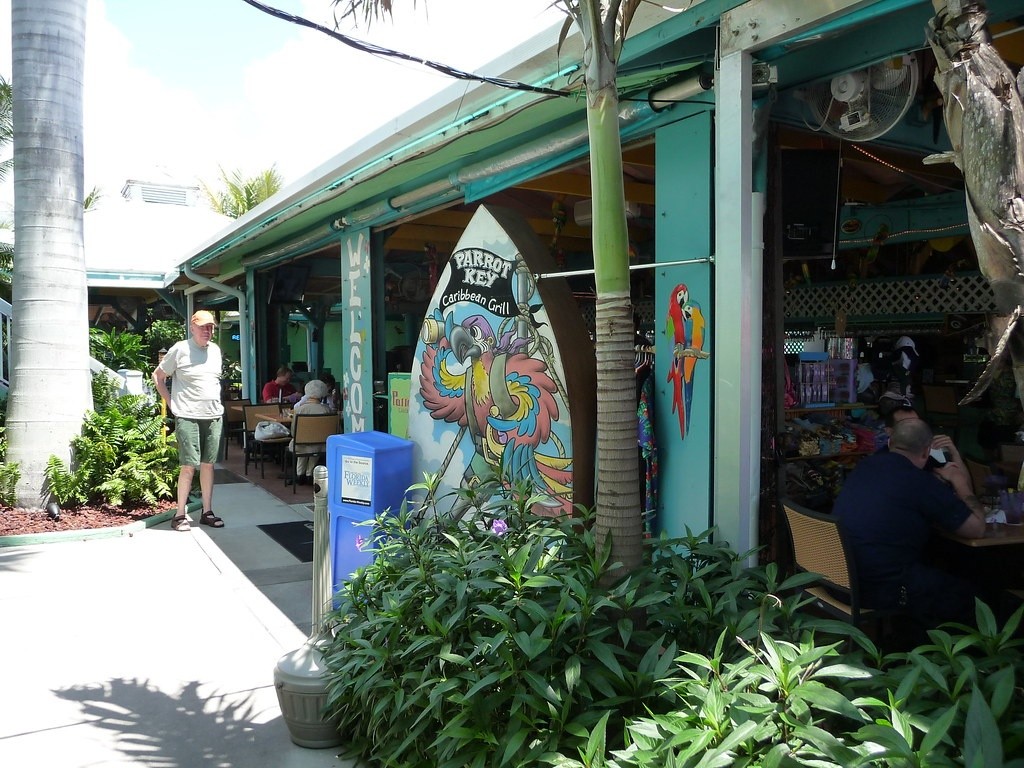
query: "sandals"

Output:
[170,512,192,532]
[199,508,224,527]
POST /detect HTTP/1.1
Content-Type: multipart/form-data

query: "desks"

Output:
[256,412,343,424]
[930,502,1024,548]
[996,461,1023,473]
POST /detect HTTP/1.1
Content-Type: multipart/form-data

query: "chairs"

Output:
[922,384,979,446]
[224,400,343,494]
[965,441,1024,496]
[777,496,906,653]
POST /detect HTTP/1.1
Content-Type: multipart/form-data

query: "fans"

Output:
[793,53,919,142]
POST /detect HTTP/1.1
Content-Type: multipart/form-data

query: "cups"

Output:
[374,381,384,392]
[999,490,1024,524]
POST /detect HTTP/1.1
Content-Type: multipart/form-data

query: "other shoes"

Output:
[295,474,306,486]
[305,474,314,486]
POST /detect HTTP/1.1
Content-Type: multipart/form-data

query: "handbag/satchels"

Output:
[255,419,291,440]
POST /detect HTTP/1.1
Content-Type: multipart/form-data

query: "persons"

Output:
[289,372,336,484]
[262,366,297,402]
[152,311,225,531]
[828,405,986,622]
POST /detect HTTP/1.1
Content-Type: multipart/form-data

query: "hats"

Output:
[190,309,219,329]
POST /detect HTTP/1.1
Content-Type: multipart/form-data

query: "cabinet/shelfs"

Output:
[785,403,879,461]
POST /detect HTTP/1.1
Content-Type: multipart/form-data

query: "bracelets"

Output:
[962,495,984,510]
